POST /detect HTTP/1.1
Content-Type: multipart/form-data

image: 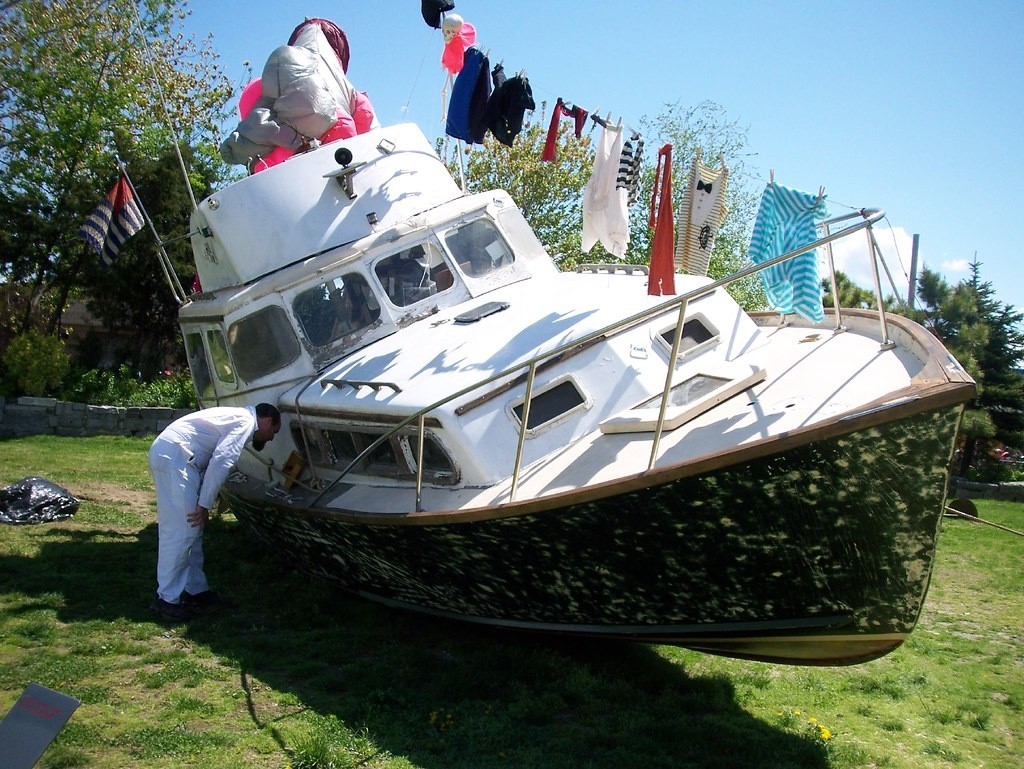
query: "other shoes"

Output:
[181,590,229,609]
[160,599,194,622]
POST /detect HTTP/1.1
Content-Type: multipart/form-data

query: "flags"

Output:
[75,174,146,272]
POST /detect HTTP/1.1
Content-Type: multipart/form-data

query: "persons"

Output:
[148,402,282,623]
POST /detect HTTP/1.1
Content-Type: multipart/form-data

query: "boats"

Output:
[173,122,982,671]
